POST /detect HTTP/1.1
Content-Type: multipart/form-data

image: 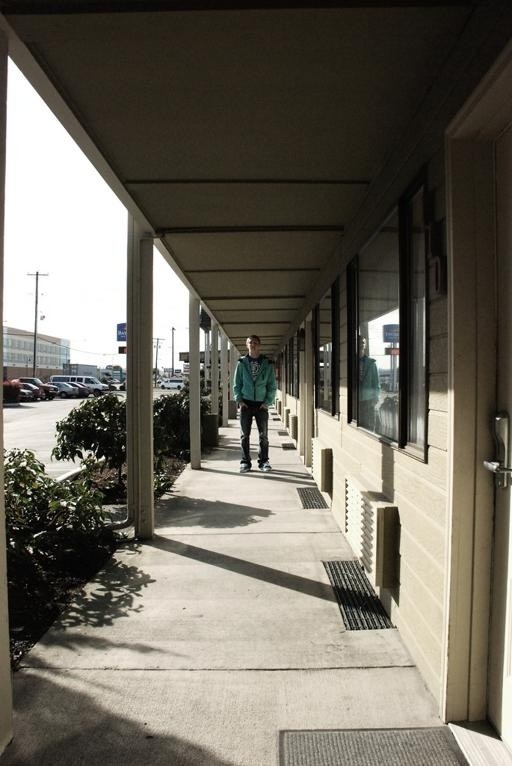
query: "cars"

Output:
[9,373,189,400]
[377,371,400,394]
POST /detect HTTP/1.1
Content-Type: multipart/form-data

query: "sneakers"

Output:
[260,465,272,473]
[240,468,251,474]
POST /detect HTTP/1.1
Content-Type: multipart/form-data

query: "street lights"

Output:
[170,326,175,378]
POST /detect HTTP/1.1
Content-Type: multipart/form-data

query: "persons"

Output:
[233,335,277,473]
[358,335,380,431]
